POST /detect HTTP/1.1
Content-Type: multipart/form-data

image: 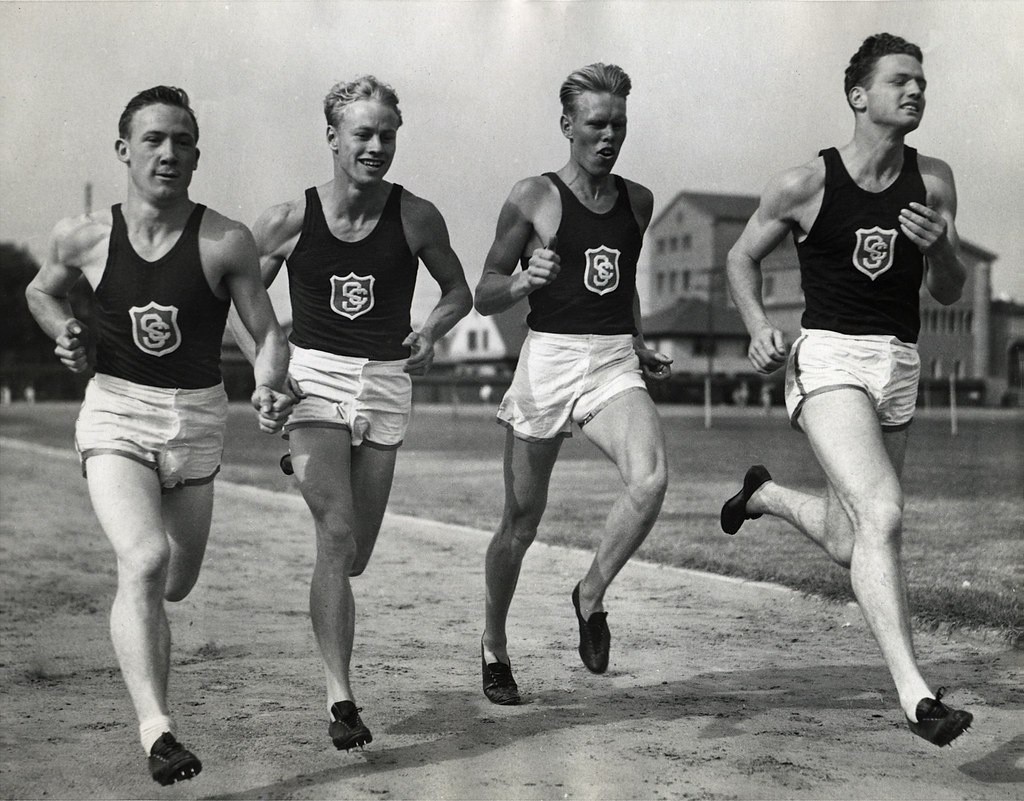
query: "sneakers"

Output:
[281,454,296,476]
[571,579,611,675]
[481,629,521,707]
[328,699,373,753]
[905,686,972,747]
[721,464,771,535]
[147,731,202,785]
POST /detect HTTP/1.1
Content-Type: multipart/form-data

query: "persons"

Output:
[473,63,675,703]
[23,85,289,788]
[721,31,977,746]
[228,75,472,752]
